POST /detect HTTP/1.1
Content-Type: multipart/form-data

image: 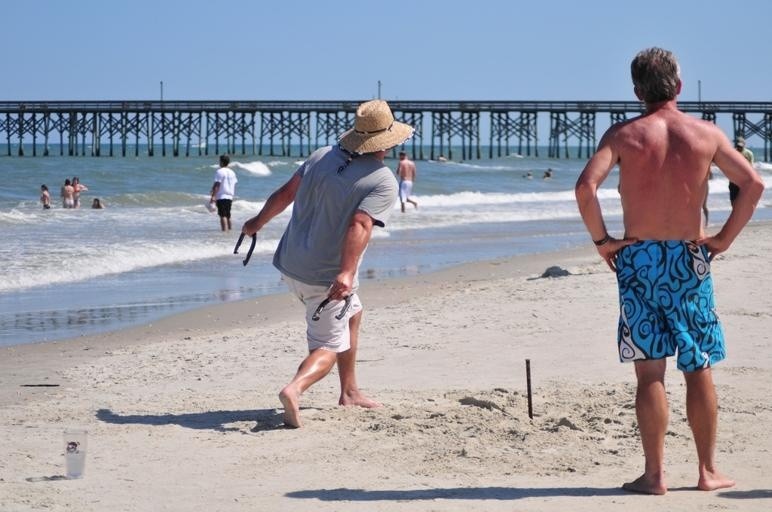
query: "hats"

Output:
[337,96,415,154]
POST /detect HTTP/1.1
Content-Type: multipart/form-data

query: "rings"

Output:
[612,257,616,261]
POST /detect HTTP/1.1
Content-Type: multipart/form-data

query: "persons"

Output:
[527,167,554,181]
[210,155,238,232]
[61,178,75,208]
[575,46,765,494]
[241,99,419,429]
[71,176,88,209]
[728,136,754,210]
[40,184,50,209]
[91,198,106,208]
[397,151,418,214]
[702,167,713,227]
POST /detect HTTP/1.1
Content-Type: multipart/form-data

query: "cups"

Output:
[64,430,87,481]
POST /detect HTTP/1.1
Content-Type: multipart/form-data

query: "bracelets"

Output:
[592,233,610,245]
